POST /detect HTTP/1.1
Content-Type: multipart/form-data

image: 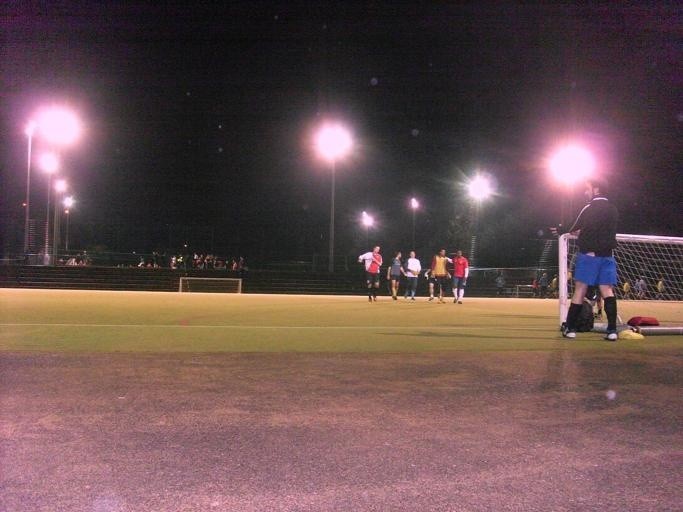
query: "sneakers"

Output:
[607,333,618,340]
[563,325,575,338]
[405,296,414,300]
[369,296,376,302]
[429,297,462,304]
[392,296,397,300]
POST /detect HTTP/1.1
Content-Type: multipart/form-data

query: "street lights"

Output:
[551,146,594,228]
[24,104,79,265]
[364,215,372,252]
[469,178,489,269]
[412,199,415,252]
[319,127,351,272]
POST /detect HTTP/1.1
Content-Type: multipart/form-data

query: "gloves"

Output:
[549,224,569,236]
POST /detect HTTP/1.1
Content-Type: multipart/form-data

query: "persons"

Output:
[358,245,383,301]
[428,247,449,301]
[404,250,422,300]
[4,248,93,266]
[534,271,667,300]
[447,250,470,303]
[387,250,407,301]
[129,244,248,272]
[556,176,618,340]
[495,274,505,295]
